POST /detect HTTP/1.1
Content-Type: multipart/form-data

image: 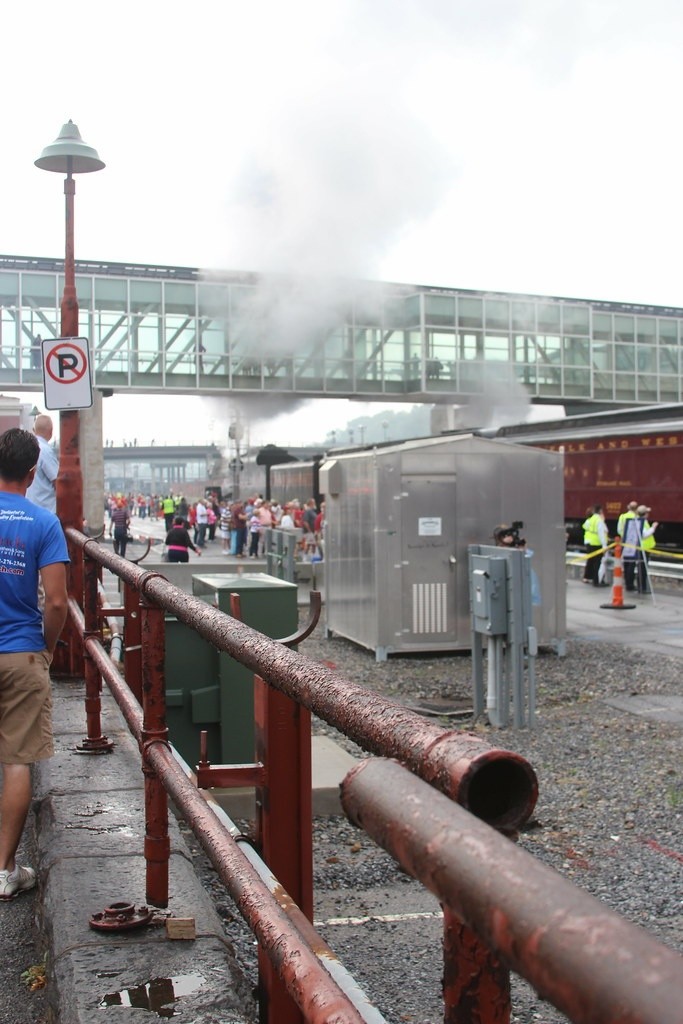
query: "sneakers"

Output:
[0,864,37,901]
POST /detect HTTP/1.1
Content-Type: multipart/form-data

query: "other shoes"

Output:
[594,583,610,587]
[583,578,593,584]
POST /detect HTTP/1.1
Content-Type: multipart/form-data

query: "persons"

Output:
[581,501,658,595]
[109,503,131,558]
[0,428,69,902]
[103,488,325,563]
[25,415,59,515]
[165,518,201,563]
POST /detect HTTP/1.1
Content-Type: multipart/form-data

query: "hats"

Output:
[637,505,652,514]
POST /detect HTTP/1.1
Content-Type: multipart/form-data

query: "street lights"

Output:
[30,121,104,677]
